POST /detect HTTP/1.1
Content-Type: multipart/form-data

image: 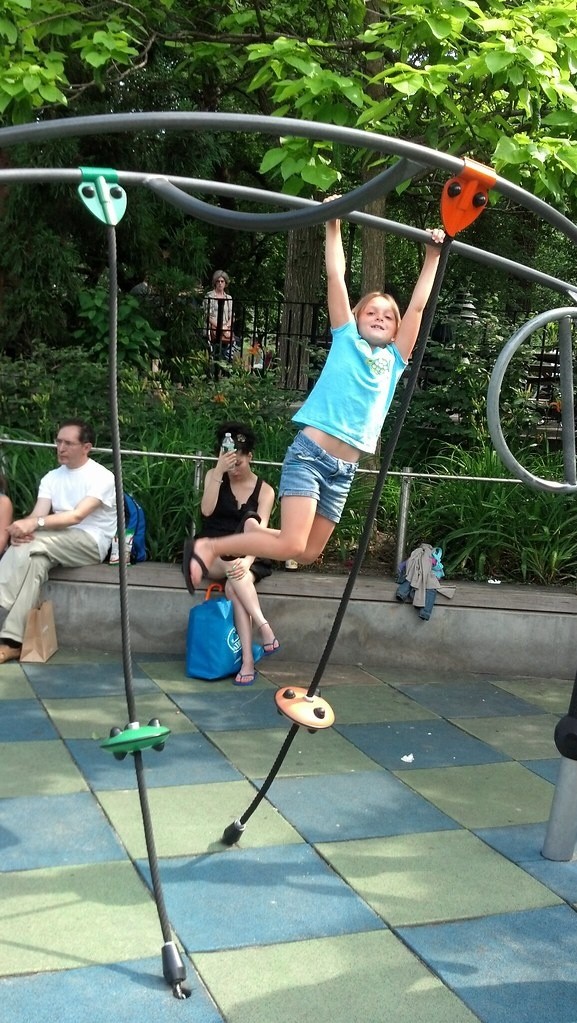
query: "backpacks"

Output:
[122,491,151,565]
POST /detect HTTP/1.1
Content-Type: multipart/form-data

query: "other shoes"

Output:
[0,645,21,663]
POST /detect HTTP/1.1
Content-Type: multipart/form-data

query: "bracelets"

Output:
[213,478,223,483]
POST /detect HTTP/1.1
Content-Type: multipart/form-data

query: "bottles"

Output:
[222,433,235,470]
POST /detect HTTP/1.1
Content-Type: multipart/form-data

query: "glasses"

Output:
[52,438,81,447]
[236,434,247,443]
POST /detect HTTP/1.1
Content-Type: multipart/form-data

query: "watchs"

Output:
[37,516,45,527]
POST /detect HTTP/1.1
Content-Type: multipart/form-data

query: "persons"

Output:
[195,421,280,686]
[181,195,445,597]
[0,419,117,663]
[0,471,12,556]
[250,337,264,371]
[203,269,236,370]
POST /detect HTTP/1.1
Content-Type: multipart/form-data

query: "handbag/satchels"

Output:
[224,341,241,360]
[252,361,263,369]
[19,588,59,664]
[185,582,264,679]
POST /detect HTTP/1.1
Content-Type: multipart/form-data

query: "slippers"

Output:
[262,635,282,655]
[232,670,259,686]
[231,510,261,558]
[182,535,209,596]
